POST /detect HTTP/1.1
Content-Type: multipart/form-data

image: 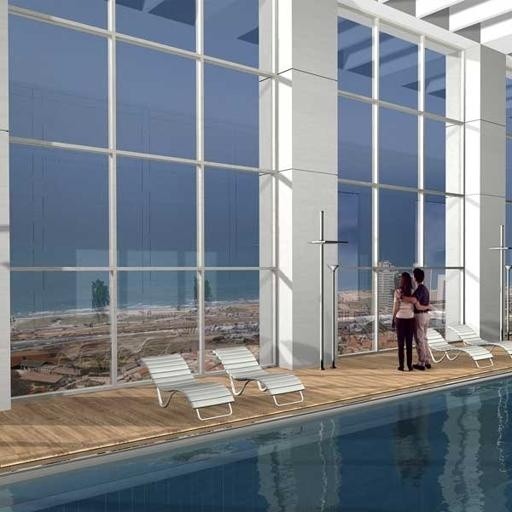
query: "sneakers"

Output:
[398,366,413,371]
[412,362,431,370]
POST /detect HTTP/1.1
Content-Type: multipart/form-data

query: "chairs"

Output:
[139,352,236,421]
[425,327,493,369]
[215,346,305,407]
[57,339,107,369]
[447,324,512,361]
[119,336,167,364]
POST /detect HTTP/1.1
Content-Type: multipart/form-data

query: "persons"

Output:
[397,268,431,371]
[392,272,432,371]
